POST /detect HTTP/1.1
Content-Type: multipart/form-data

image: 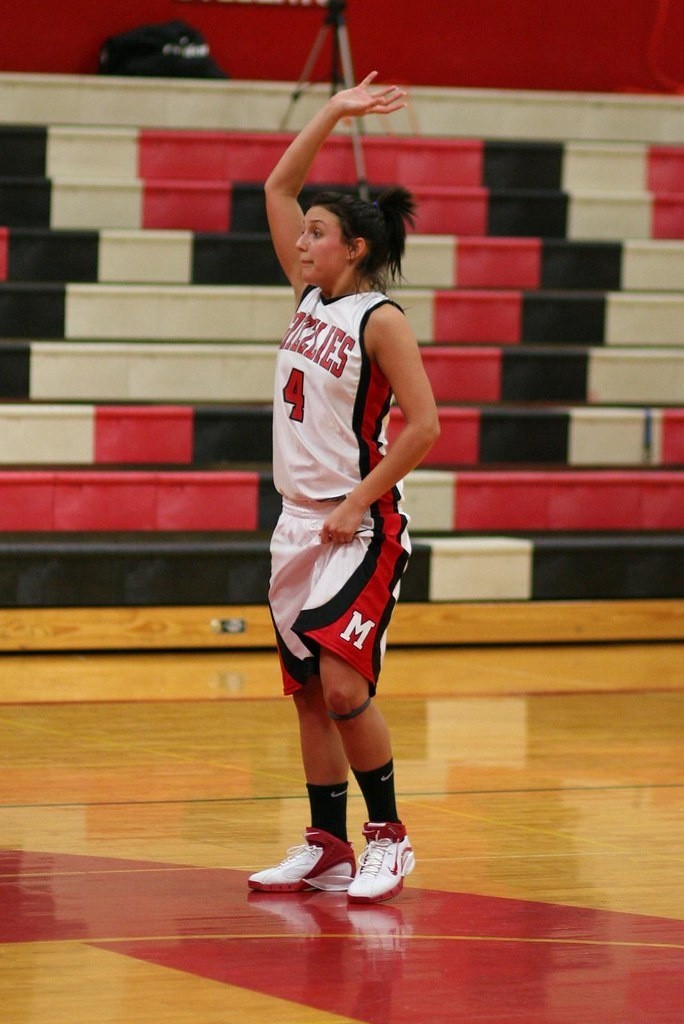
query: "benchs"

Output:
[0,73,684,705]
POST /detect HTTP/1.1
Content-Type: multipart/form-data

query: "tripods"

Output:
[284,0,368,197]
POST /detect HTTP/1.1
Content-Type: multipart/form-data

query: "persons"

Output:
[247,72,441,907]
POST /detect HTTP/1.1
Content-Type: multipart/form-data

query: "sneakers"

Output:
[247,826,356,892]
[347,821,415,903]
